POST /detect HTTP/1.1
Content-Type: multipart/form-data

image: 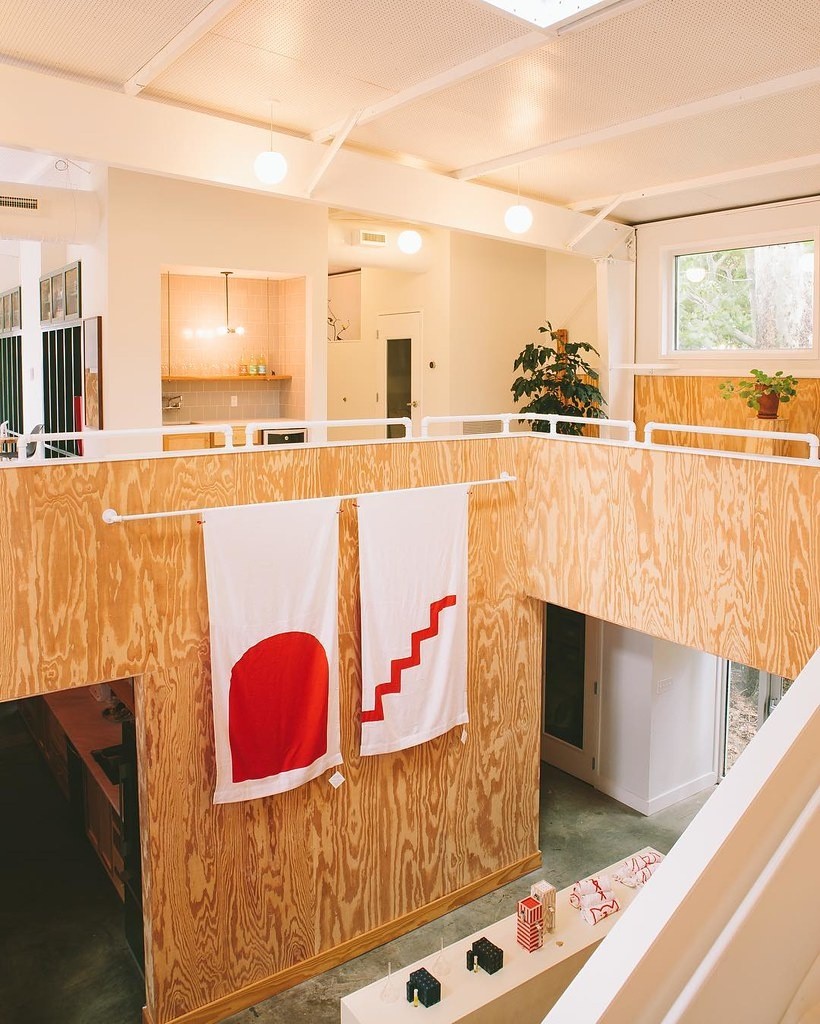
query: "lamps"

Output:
[256,98,286,185]
[217,272,244,336]
[505,165,532,234]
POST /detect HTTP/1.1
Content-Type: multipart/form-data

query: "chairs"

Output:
[0,424,44,462]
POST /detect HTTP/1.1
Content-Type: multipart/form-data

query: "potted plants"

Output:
[717,369,800,419]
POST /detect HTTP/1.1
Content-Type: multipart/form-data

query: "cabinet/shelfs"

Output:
[31,679,124,904]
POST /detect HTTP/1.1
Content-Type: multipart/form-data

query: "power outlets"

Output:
[231,396,238,407]
